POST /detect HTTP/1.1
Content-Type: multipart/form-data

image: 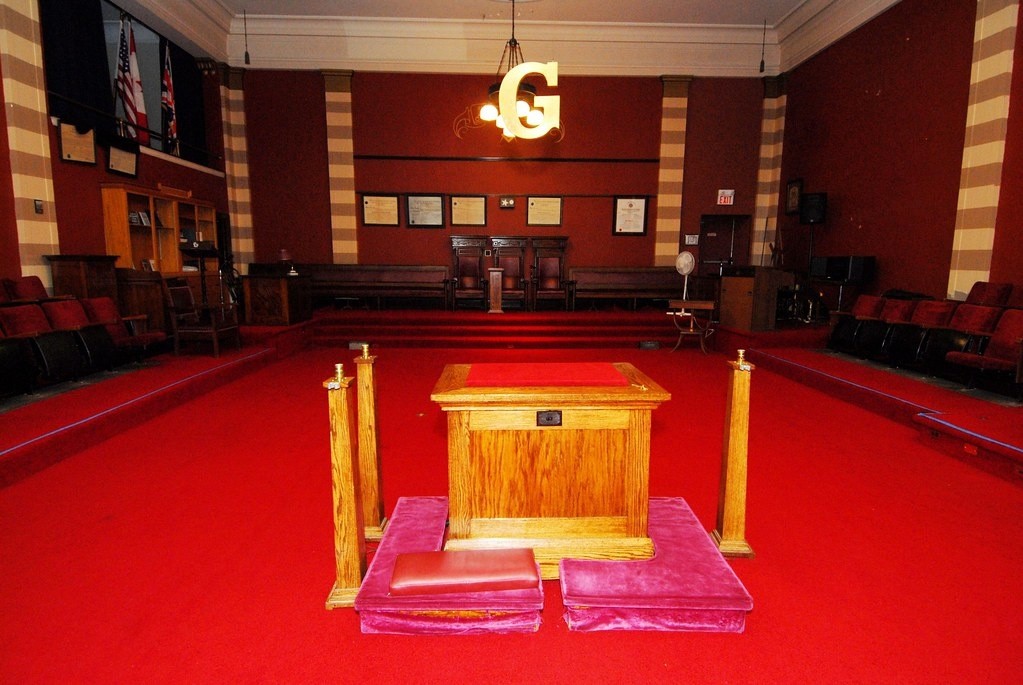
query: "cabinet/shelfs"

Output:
[101,183,218,272]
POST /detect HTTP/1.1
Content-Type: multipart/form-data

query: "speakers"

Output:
[798,193,829,224]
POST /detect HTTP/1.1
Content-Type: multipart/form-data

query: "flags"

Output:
[129,21,152,149]
[113,17,138,141]
[161,46,181,157]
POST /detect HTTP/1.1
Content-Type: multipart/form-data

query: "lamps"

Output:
[454,0,565,145]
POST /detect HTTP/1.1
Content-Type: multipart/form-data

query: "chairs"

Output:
[0,275,241,380]
[530,236,570,309]
[810,256,1023,390]
[450,235,489,311]
[491,236,528,313]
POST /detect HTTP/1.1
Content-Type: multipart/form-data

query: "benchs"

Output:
[568,267,684,310]
[248,262,450,311]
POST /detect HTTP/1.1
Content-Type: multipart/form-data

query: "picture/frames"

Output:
[785,177,804,216]
[58,119,97,166]
[612,194,648,236]
[450,193,487,226]
[106,145,139,179]
[404,193,446,228]
[526,195,564,226]
[361,194,400,226]
